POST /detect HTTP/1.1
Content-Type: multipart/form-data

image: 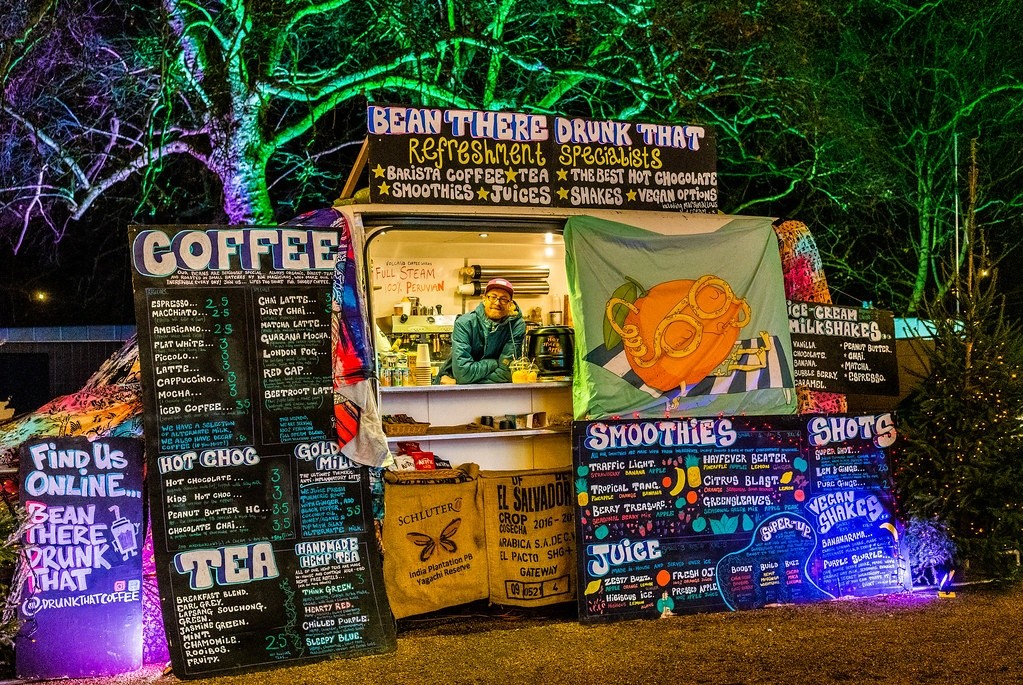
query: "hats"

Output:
[486,279,514,299]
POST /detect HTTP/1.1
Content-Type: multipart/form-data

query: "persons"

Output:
[433,279,525,385]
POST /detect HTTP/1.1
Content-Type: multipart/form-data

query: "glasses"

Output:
[486,294,511,305]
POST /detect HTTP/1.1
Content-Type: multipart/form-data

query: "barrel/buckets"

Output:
[527,326,574,375]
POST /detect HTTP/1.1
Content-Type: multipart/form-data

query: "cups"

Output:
[394,307,403,316]
[457,284,474,295]
[549,311,562,325]
[415,344,431,385]
[508,361,539,383]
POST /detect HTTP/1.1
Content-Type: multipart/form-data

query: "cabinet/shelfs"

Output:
[379,378,577,441]
